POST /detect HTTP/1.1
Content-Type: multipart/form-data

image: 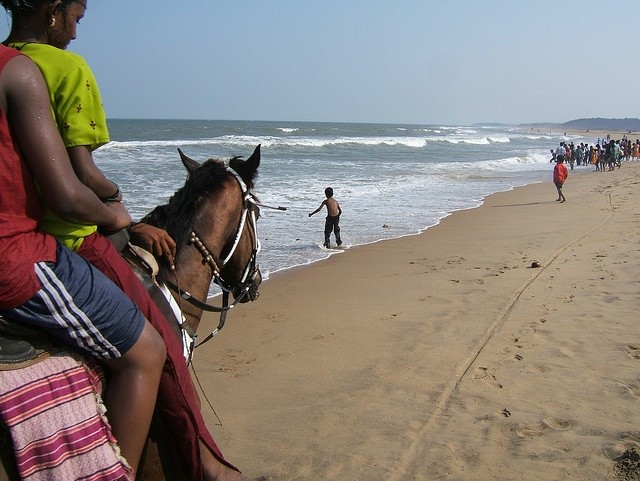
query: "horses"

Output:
[0,143,263,481]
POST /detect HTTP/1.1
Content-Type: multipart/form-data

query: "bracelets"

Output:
[104,179,122,202]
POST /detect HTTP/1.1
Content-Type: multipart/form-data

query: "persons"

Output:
[550,141,567,164]
[553,155,568,203]
[308,188,343,248]
[0,0,268,480]
[1,45,167,480]
[563,134,640,172]
